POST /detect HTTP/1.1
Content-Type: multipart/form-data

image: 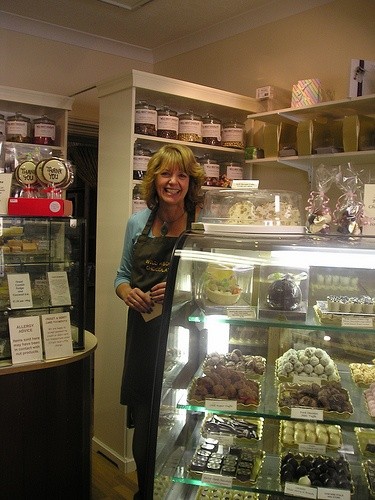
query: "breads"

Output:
[275,346,353,413]
[201,414,259,440]
[223,200,301,227]
[188,438,256,482]
[189,349,265,405]
[350,358,375,417]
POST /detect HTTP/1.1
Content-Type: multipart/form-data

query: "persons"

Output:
[114,144,205,500]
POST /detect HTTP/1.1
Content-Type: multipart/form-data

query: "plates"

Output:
[195,485,259,500]
[186,376,261,407]
[185,445,265,483]
[361,460,375,498]
[275,359,341,383]
[276,383,354,415]
[202,354,266,377]
[354,427,375,456]
[279,420,342,449]
[279,452,355,495]
[363,389,375,418]
[313,300,375,327]
[159,405,178,433]
[349,363,375,388]
[201,412,264,442]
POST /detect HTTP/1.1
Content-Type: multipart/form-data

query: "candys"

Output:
[14,157,74,199]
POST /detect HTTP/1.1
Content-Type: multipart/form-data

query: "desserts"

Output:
[362,443,375,495]
[198,488,256,500]
[280,420,352,493]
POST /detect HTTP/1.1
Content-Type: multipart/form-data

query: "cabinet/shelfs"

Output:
[0,71,375,500]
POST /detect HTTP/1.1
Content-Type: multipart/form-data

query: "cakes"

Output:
[203,276,242,305]
[326,295,375,314]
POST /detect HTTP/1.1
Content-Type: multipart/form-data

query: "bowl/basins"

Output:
[206,283,240,304]
